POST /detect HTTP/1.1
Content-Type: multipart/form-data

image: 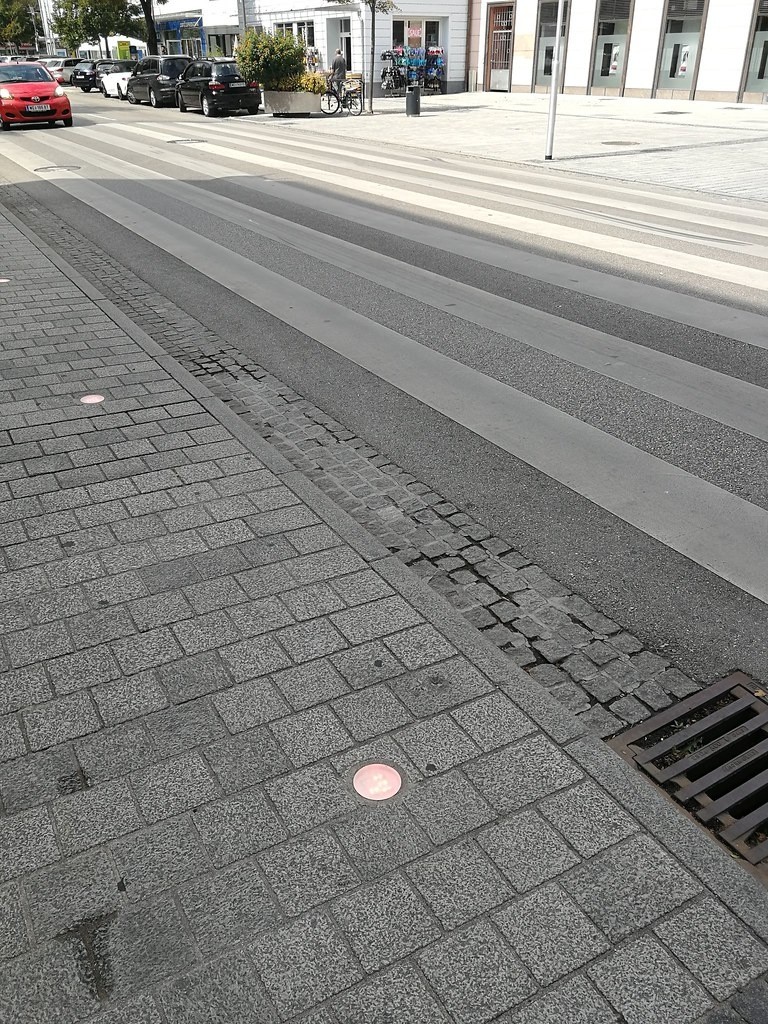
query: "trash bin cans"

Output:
[405,85,420,117]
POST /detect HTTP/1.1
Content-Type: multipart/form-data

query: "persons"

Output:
[330,48,347,95]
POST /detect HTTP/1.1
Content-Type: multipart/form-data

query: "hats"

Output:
[335,49,341,54]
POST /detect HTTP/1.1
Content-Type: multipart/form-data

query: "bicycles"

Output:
[320,74,364,117]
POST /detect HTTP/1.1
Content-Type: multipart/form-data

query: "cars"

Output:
[72,58,109,93]
[0,55,85,87]
[0,61,73,131]
[95,59,136,100]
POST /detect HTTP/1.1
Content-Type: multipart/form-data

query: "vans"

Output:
[174,56,263,117]
[126,54,193,109]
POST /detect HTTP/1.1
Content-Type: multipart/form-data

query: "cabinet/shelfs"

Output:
[380,46,444,97]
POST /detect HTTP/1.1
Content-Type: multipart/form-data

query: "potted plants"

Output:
[264,72,328,114]
